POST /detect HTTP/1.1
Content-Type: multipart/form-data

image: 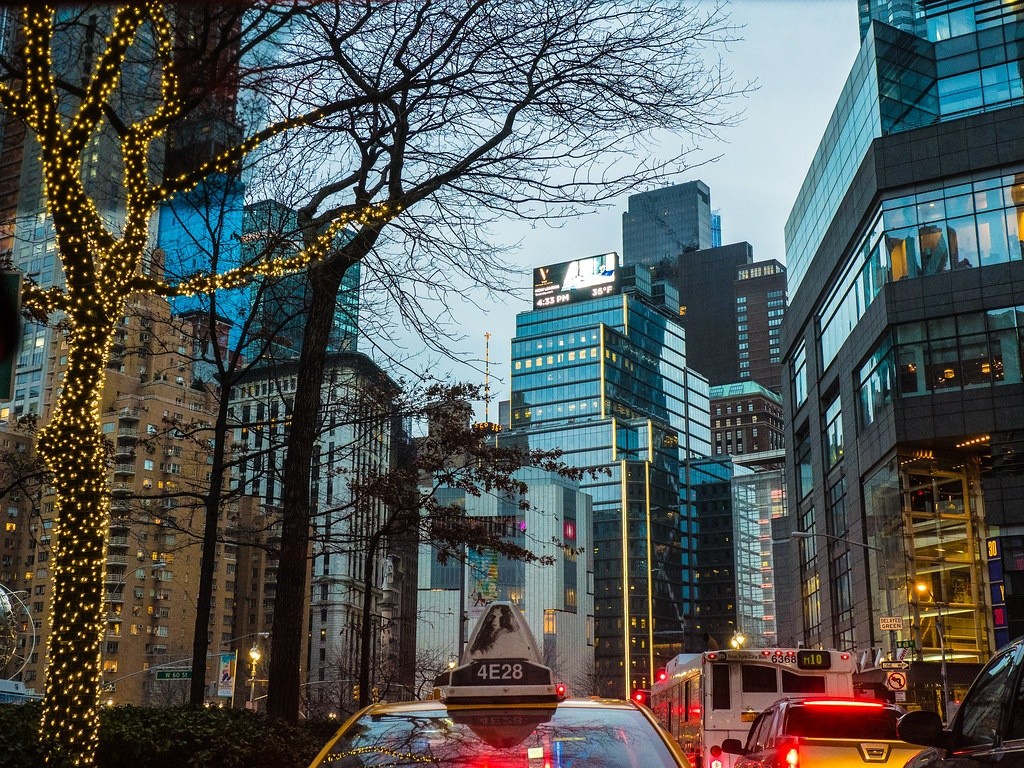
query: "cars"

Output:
[720,695,932,768]
[895,634,1023,768]
[308,696,692,767]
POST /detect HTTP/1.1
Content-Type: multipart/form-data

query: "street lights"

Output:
[916,582,941,627]
[249,642,261,710]
[791,531,897,662]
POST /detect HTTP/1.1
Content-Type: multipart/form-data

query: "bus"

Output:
[650,648,854,768]
